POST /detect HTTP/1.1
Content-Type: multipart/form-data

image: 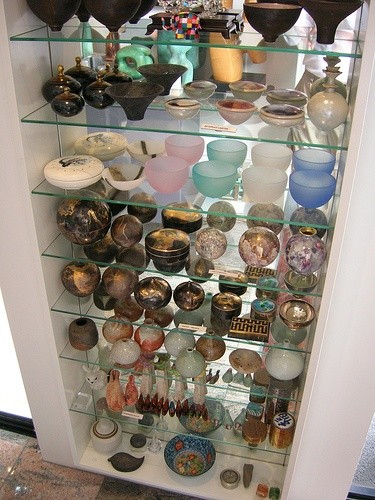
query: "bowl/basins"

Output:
[257,0,297,4]
[297,0,364,44]
[178,396,225,433]
[242,165,287,204]
[293,149,336,174]
[207,139,247,170]
[284,270,318,298]
[289,169,337,207]
[243,3,302,42]
[165,135,204,165]
[105,82,164,121]
[192,160,237,198]
[75,0,92,22]
[144,156,189,194]
[163,433,216,477]
[137,64,187,96]
[26,0,81,31]
[102,164,147,191]
[129,0,158,24]
[126,140,165,162]
[82,0,142,32]
[251,143,292,171]
[279,300,316,329]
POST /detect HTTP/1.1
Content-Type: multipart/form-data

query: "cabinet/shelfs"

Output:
[0,0,375,500]
